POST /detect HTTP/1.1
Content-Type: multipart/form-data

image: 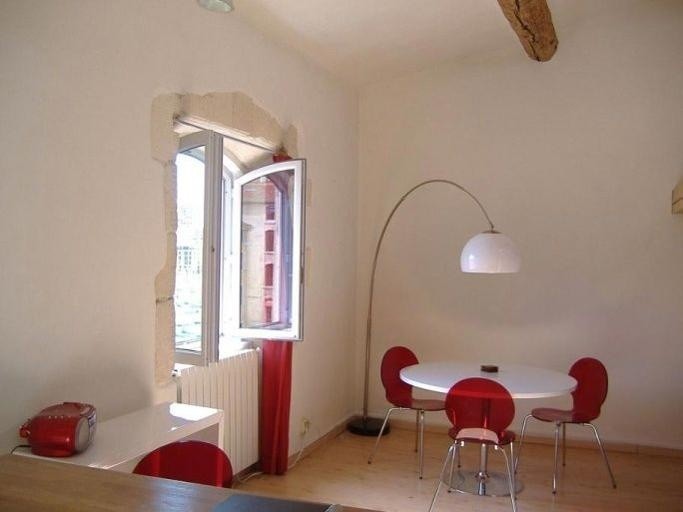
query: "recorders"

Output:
[20,402,96,457]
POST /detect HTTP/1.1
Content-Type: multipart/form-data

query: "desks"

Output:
[0,401,377,512]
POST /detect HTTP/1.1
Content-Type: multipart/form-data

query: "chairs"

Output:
[513,357,617,496]
[367,345,461,480]
[430,376,517,512]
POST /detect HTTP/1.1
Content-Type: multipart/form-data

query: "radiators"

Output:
[174,348,260,478]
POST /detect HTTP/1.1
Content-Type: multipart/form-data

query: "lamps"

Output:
[346,178,523,435]
